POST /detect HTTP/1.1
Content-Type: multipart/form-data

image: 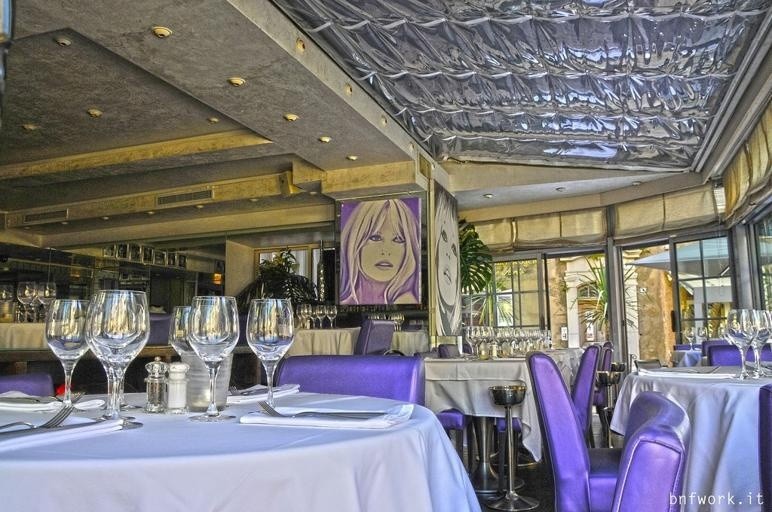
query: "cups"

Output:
[169,305,196,357]
[180,352,235,413]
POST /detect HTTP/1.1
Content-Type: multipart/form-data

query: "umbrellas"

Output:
[624,233,772,277]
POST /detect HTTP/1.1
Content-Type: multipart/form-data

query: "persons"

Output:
[433,185,465,338]
[338,198,421,305]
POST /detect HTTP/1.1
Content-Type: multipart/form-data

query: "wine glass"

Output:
[0,279,60,323]
[683,324,724,352]
[293,304,339,330]
[724,309,772,380]
[367,312,405,333]
[187,295,240,423]
[46,299,91,413]
[85,289,151,433]
[245,299,296,415]
[463,326,552,361]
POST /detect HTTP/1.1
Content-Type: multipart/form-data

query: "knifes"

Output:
[0,390,86,404]
[0,421,105,441]
[230,386,282,396]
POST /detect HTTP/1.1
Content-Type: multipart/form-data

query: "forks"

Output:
[259,400,389,420]
[647,365,721,375]
[0,404,75,429]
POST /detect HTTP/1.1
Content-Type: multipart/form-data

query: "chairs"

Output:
[0,314,769,512]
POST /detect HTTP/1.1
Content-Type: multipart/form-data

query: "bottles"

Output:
[167,362,189,415]
[145,356,168,414]
